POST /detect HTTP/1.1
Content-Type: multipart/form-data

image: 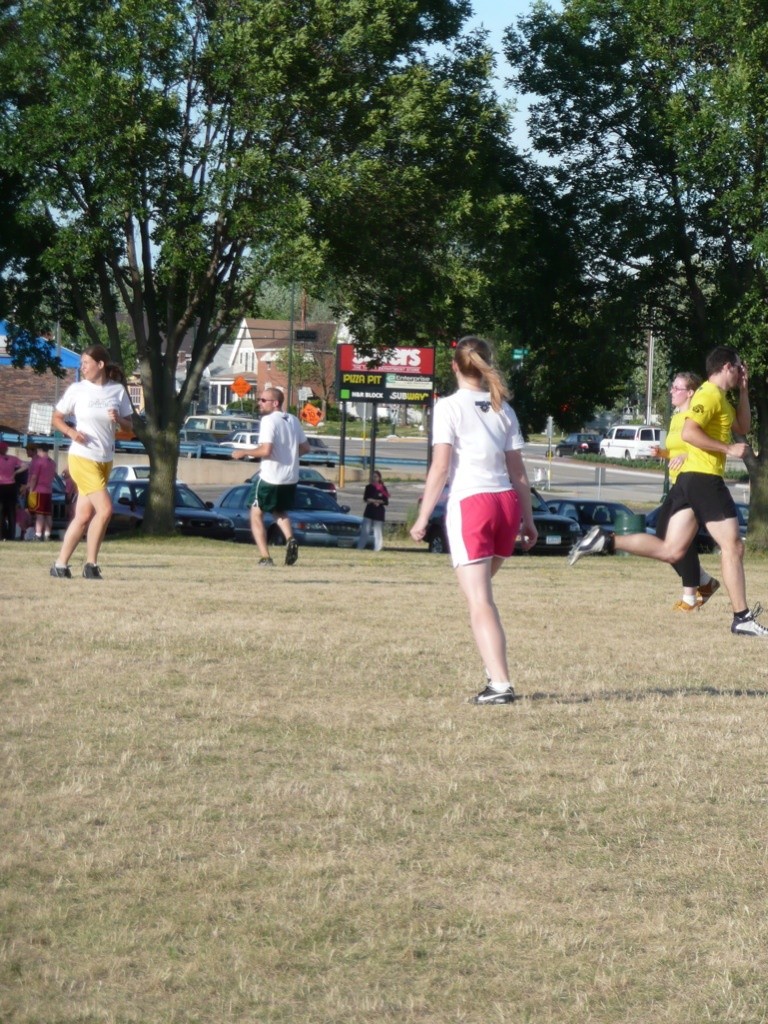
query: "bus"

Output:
[182,415,260,433]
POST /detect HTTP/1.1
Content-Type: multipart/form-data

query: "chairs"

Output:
[595,511,608,520]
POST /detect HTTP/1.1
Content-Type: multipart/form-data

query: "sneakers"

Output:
[731,602,768,635]
[466,685,513,705]
[569,525,612,566]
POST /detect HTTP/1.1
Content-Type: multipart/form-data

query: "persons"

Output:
[356,470,389,552]
[649,371,720,612]
[232,386,310,567]
[568,346,768,635]
[0,442,78,543]
[408,335,538,704]
[51,347,134,577]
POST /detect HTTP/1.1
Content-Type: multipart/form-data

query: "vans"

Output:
[597,425,666,464]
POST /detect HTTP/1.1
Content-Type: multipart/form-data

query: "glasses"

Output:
[257,397,276,403]
[670,385,691,392]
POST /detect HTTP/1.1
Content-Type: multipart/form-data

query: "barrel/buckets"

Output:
[614,514,646,558]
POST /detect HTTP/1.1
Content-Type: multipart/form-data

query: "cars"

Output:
[177,429,220,460]
[245,467,336,502]
[13,464,222,534]
[539,498,655,539]
[419,487,582,553]
[637,503,750,553]
[220,479,374,550]
[555,431,600,456]
[223,431,339,468]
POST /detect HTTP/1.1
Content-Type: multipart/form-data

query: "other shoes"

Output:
[83,563,102,579]
[259,558,273,566]
[32,534,51,541]
[671,578,721,613]
[285,537,298,565]
[50,566,71,579]
